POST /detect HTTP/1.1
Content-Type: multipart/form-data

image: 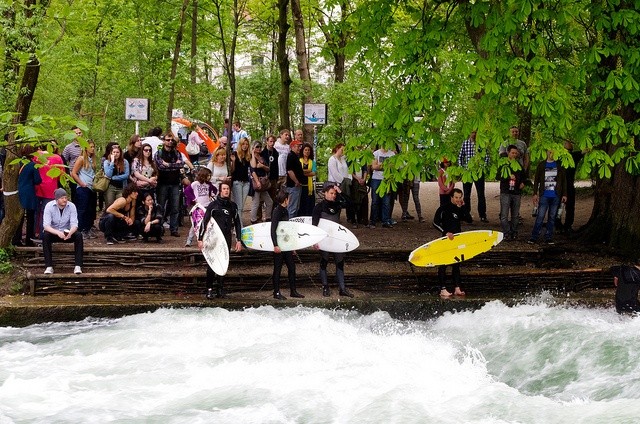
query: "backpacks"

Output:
[621,265,640,283]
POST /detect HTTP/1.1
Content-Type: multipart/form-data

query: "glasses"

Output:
[112,145,120,148]
[472,131,476,134]
[143,149,152,152]
[216,147,225,151]
[76,132,82,134]
[87,139,92,141]
[565,148,574,152]
[222,142,227,145]
[164,139,174,142]
[511,152,518,154]
[548,150,551,153]
[256,146,262,148]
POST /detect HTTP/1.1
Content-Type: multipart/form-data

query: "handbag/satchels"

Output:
[248,179,255,196]
[252,176,271,191]
[186,141,200,155]
[323,181,336,190]
[92,170,110,191]
[200,145,208,155]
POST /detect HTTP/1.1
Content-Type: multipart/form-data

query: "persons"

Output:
[31,140,65,238]
[50,140,67,188]
[231,137,256,234]
[298,143,317,217]
[198,182,241,299]
[251,141,274,224]
[526,148,567,244]
[555,143,590,231]
[207,146,236,199]
[286,140,304,218]
[312,185,355,299]
[137,193,165,241]
[100,142,119,168]
[230,122,248,153]
[103,145,130,208]
[99,182,139,245]
[12,145,42,247]
[219,136,228,148]
[496,144,526,240]
[223,118,235,137]
[272,191,306,299]
[71,139,98,239]
[433,188,473,297]
[498,125,529,219]
[273,129,291,185]
[437,158,455,206]
[175,133,191,162]
[184,167,217,247]
[328,143,426,228]
[141,127,163,159]
[124,134,142,163]
[62,126,85,204]
[0,133,11,226]
[261,135,279,200]
[131,143,158,241]
[180,152,194,215]
[458,129,490,223]
[185,122,205,163]
[42,187,83,274]
[613,260,640,314]
[155,134,183,237]
[290,129,303,148]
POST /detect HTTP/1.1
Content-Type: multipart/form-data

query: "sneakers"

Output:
[348,222,358,228]
[81,232,89,239]
[139,235,143,239]
[217,289,228,298]
[290,286,305,298]
[27,240,40,247]
[419,217,425,222]
[528,239,535,244]
[564,224,573,230]
[185,240,191,246]
[517,215,523,224]
[401,212,407,222]
[359,222,369,228]
[556,224,562,231]
[262,219,271,222]
[368,223,375,228]
[207,288,213,299]
[143,236,149,243]
[44,267,54,274]
[505,234,511,241]
[511,232,518,239]
[382,224,392,229]
[113,238,126,243]
[12,238,25,247]
[107,238,113,244]
[480,217,489,223]
[74,266,82,274]
[323,284,330,296]
[406,212,414,220]
[548,240,554,244]
[273,290,287,300]
[171,233,180,237]
[251,221,259,224]
[387,219,397,224]
[128,236,137,241]
[87,229,97,238]
[338,288,349,296]
[157,237,162,243]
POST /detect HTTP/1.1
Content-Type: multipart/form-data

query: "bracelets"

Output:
[120,215,126,220]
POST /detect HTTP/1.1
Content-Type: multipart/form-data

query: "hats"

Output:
[200,165,212,173]
[444,160,449,162]
[290,140,303,148]
[54,188,67,200]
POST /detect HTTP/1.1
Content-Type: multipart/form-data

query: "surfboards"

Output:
[233,221,328,251]
[289,216,360,253]
[408,230,504,268]
[189,203,230,277]
